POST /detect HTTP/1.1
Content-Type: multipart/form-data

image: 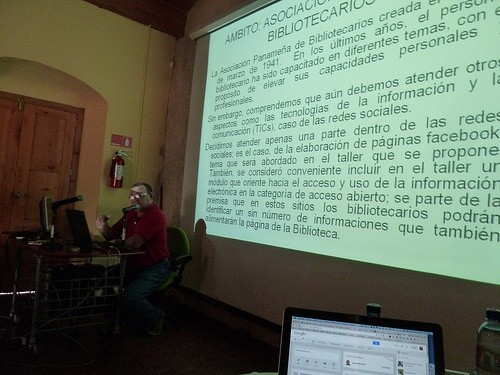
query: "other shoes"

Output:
[148,309,165,335]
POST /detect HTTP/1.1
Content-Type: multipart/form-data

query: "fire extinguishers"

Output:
[109,150,127,189]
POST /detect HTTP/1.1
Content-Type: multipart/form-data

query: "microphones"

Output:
[53,195,85,208]
[123,204,141,213]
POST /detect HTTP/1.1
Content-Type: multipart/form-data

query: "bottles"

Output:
[365,303,382,318]
[472,307,500,375]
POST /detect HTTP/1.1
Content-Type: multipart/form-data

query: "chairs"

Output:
[157,226,193,330]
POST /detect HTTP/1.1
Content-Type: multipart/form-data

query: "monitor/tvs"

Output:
[39,196,55,238]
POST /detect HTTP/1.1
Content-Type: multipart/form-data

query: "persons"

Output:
[95,181,172,337]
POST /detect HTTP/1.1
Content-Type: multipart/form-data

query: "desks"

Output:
[4,235,147,358]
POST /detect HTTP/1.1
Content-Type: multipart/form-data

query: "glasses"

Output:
[129,192,150,199]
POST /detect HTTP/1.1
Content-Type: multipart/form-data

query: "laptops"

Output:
[278,307,446,375]
[64,209,102,251]
